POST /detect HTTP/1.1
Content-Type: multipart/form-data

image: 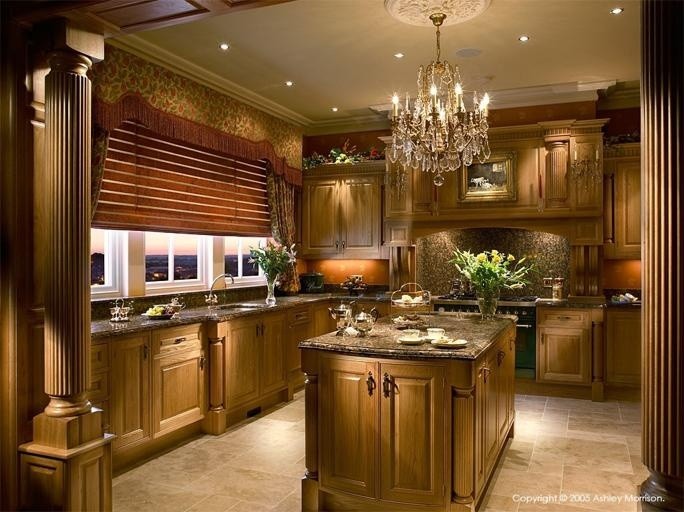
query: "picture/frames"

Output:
[458,150,517,204]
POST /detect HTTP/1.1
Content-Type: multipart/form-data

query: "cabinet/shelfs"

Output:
[313,355,454,508]
[101,331,152,447]
[536,303,595,390]
[300,162,382,259]
[225,312,296,425]
[152,322,211,439]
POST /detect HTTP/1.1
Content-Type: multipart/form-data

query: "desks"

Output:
[298,311,520,512]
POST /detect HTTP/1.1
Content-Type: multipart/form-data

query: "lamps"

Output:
[286,81,293,87]
[387,59,489,187]
[220,43,229,50]
[394,53,404,59]
[609,8,623,15]
[572,149,605,193]
[517,36,532,42]
[332,107,338,112]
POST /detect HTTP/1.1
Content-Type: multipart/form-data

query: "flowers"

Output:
[249,236,297,296]
[450,245,539,312]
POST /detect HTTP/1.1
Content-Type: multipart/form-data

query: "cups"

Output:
[428,328,445,339]
[109,307,130,320]
[404,328,420,339]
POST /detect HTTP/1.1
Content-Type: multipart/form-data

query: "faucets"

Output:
[204,274,234,308]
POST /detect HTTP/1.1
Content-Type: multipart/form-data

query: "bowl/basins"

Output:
[153,305,181,312]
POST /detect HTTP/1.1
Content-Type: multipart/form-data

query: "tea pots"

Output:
[348,308,379,338]
[328,300,357,337]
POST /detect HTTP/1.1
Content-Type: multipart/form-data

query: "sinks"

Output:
[221,304,265,310]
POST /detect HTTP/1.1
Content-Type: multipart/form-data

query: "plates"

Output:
[431,339,468,349]
[141,313,174,320]
[422,336,449,343]
[400,339,424,345]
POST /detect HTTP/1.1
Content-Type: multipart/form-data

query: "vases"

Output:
[264,274,277,305]
[476,290,498,323]
